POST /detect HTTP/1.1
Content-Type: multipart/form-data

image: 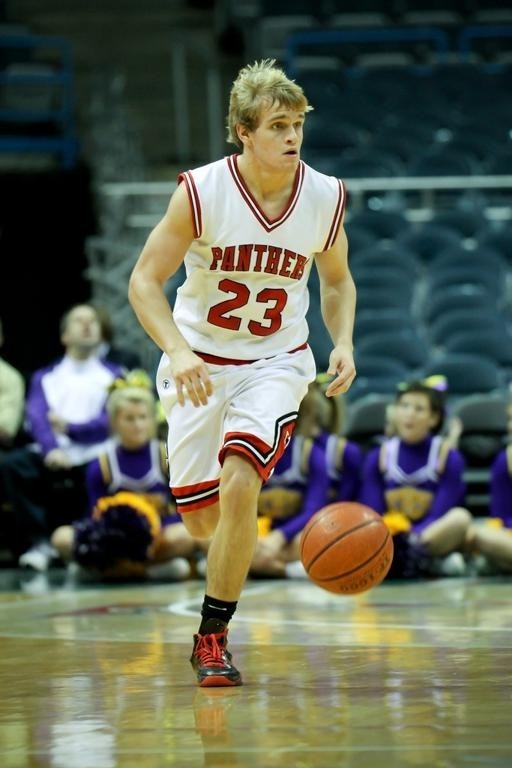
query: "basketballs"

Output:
[300,501,395,595]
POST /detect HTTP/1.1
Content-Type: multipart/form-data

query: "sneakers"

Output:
[145,553,208,580]
[189,616,245,687]
[427,553,509,577]
[17,535,62,572]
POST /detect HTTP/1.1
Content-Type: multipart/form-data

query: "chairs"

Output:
[257,0,512,513]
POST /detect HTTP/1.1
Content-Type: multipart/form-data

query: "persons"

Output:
[1,302,135,573]
[52,371,194,580]
[249,431,327,581]
[290,369,360,507]
[93,307,146,371]
[128,55,356,689]
[1,358,28,568]
[463,403,511,576]
[361,374,474,577]
